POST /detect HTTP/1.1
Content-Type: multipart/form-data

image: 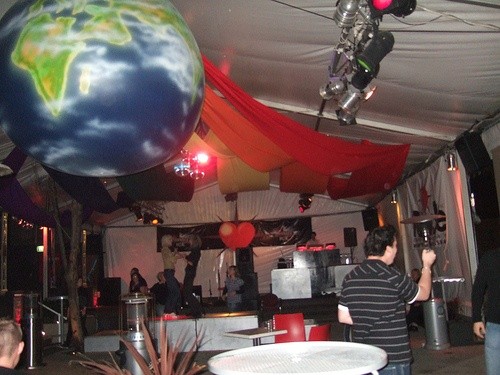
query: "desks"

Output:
[208,341,389,375]
[225,328,288,346]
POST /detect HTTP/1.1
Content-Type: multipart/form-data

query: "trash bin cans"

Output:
[422,298,453,350]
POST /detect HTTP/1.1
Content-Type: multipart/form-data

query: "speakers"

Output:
[343,227,358,247]
[191,285,202,304]
[98,277,121,306]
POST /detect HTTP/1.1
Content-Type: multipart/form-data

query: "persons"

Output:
[221,264,246,308]
[58,277,88,348]
[337,224,436,375]
[129,234,202,314]
[406,269,425,331]
[0,319,27,375]
[471,248,500,375]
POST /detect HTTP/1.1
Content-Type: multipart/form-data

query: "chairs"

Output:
[308,324,332,341]
[272,312,307,344]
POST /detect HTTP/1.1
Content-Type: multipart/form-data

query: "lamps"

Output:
[392,193,399,205]
[318,0,419,125]
[130,200,167,226]
[448,151,461,173]
[297,192,318,213]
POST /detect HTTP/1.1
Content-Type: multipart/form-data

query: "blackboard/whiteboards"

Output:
[271,268,312,299]
[329,263,362,296]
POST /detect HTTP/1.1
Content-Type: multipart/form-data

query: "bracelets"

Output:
[422,267,431,273]
